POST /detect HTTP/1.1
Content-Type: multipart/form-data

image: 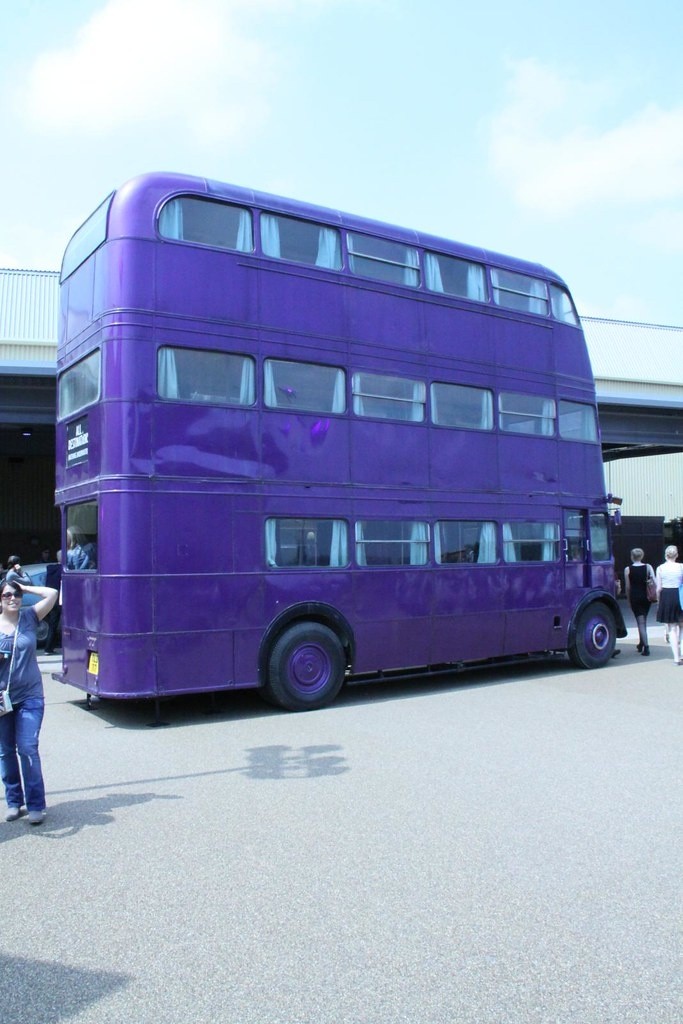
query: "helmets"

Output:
[8,556,20,563]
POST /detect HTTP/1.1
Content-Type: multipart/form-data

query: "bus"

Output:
[49,171,630,713]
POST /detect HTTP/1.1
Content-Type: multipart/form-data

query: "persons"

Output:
[0,580,59,825]
[656,545,683,665]
[6,555,32,586]
[624,548,656,656]
[43,550,62,655]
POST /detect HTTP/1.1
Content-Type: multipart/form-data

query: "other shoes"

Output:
[29,811,44,824]
[674,656,682,665]
[636,644,650,655]
[45,650,55,656]
[4,807,20,821]
[611,649,620,658]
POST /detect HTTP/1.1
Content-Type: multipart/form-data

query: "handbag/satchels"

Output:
[0,690,13,716]
[679,584,683,611]
[646,577,657,602]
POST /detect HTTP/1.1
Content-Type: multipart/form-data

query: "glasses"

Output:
[1,592,21,600]
